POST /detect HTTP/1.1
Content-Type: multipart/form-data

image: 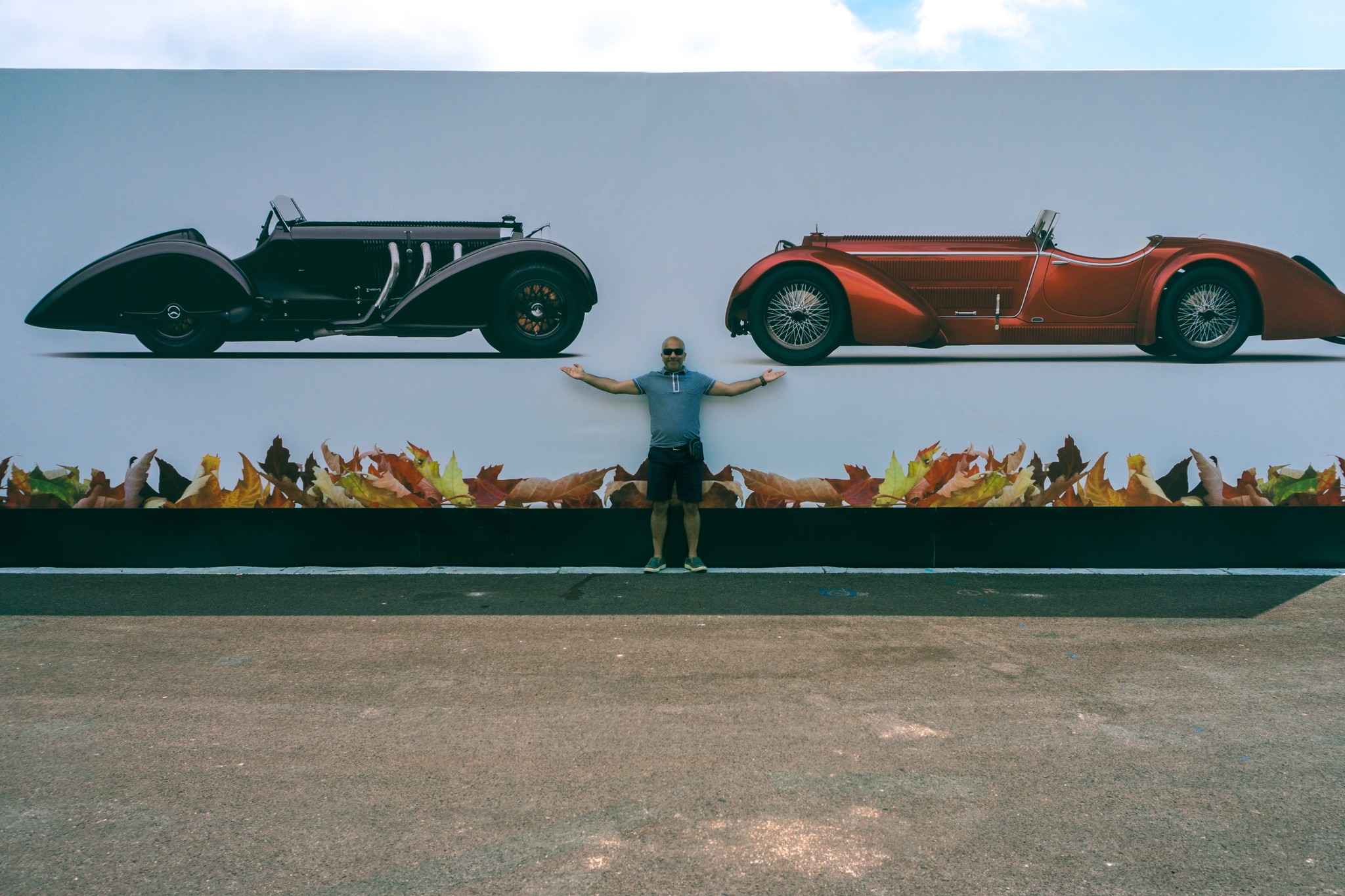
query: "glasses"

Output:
[662,348,686,355]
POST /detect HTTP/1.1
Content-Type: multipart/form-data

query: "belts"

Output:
[672,444,688,451]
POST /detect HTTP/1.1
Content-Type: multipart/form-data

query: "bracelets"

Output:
[759,375,767,386]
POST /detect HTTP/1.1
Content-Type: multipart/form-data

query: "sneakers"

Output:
[644,556,666,572]
[684,555,707,572]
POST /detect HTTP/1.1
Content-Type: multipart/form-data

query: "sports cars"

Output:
[725,208,1345,365]
[22,193,599,360]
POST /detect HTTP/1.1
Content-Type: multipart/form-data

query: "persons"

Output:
[561,336,787,573]
